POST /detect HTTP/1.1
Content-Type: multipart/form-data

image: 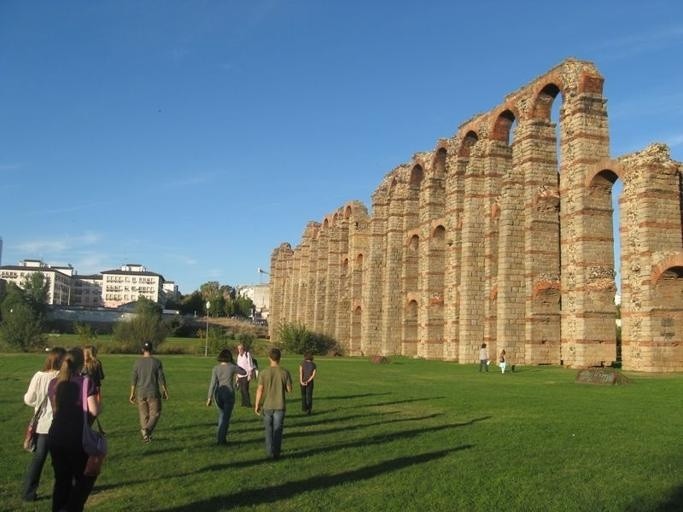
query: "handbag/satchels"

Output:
[245,350,259,370]
[23,416,37,451]
[82,429,107,456]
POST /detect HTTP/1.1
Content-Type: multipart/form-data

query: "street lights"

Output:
[205,301,211,356]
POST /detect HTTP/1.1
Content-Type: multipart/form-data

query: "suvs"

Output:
[251,319,268,328]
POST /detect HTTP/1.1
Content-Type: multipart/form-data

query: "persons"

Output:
[235,343,257,409]
[479,343,489,373]
[21,347,67,502]
[79,345,104,404]
[499,349,506,374]
[254,348,293,463]
[298,350,317,417]
[48,347,108,512]
[129,340,169,444]
[206,349,247,446]
